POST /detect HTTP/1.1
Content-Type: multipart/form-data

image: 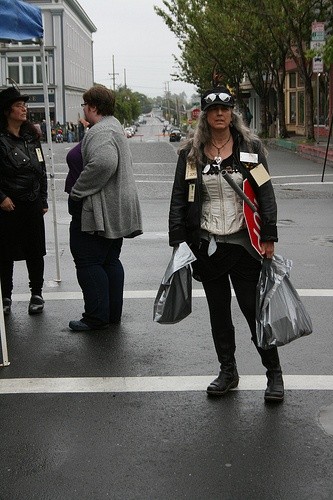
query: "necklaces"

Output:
[209,133,232,154]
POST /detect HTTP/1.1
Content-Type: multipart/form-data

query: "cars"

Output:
[124,114,185,143]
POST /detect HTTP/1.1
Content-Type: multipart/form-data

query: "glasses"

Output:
[15,105,28,110]
[81,103,88,107]
[204,92,232,104]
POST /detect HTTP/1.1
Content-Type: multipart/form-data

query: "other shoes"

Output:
[82,312,121,323]
[69,319,110,330]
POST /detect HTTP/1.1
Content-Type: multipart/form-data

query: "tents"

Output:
[0,0,61,367]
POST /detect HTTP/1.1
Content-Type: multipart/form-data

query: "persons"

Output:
[0,86,48,312]
[40,118,89,144]
[162,129,165,135]
[168,87,284,400]
[64,84,143,331]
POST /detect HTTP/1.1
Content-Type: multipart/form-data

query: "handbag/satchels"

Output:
[255,253,312,350]
[153,241,197,325]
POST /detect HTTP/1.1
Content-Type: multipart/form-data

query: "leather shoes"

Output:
[28,294,46,314]
[3,298,12,315]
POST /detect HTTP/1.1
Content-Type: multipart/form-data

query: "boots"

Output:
[206,326,240,394]
[251,336,284,401]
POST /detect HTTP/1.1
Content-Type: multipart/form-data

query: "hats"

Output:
[201,86,234,110]
[0,86,30,112]
